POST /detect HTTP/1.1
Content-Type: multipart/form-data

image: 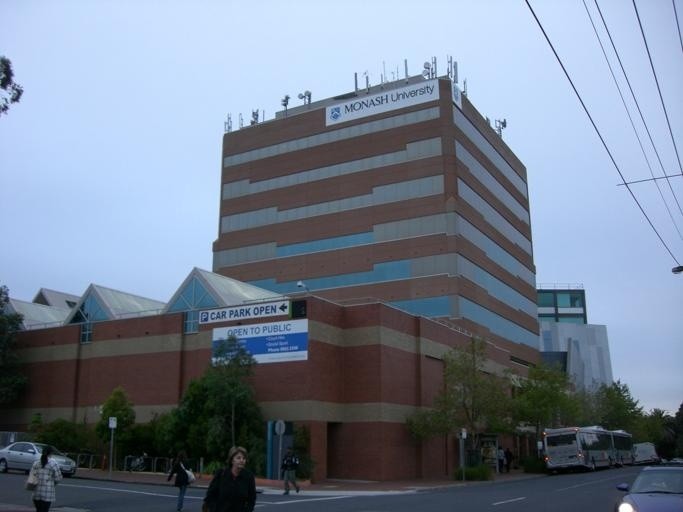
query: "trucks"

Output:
[633,442,660,465]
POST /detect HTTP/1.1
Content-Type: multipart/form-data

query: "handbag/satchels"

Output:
[186,469,196,483]
[26,472,39,491]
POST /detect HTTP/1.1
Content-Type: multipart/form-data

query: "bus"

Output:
[543,425,636,475]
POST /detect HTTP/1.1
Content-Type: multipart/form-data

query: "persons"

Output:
[167,458,189,512]
[126,455,132,472]
[202,446,257,512]
[504,448,514,472]
[280,446,300,495]
[29,446,63,512]
[497,447,505,472]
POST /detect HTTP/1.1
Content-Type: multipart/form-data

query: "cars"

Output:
[615,467,683,512]
[670,458,683,464]
[0,441,76,478]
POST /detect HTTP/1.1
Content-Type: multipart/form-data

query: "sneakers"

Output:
[284,491,289,495]
[296,487,300,492]
